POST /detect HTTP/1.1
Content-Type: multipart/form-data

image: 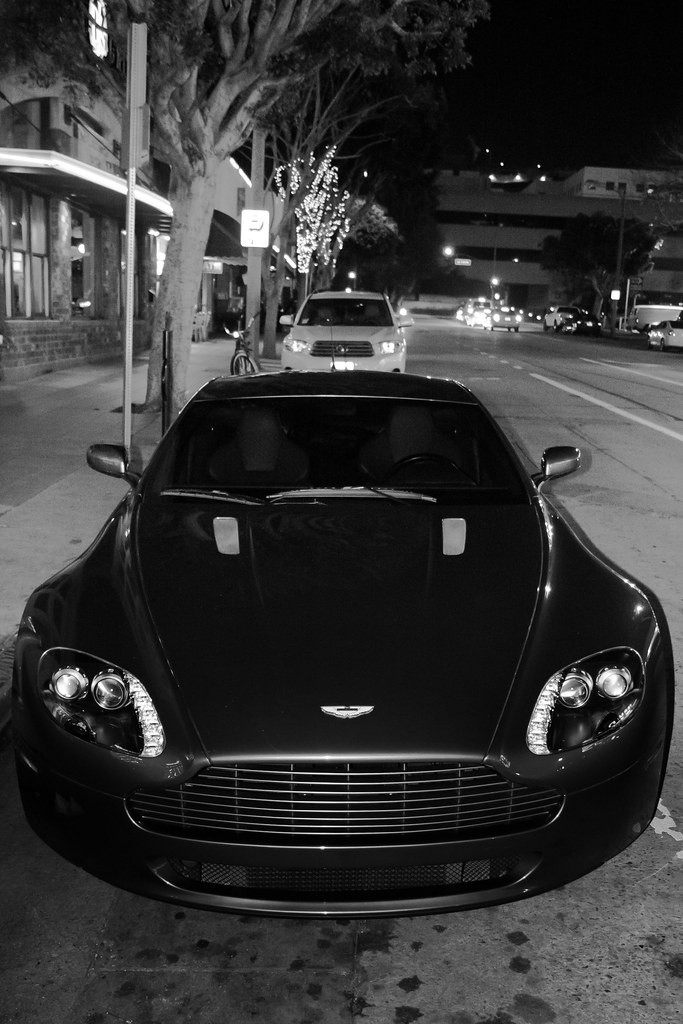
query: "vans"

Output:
[630,305,683,331]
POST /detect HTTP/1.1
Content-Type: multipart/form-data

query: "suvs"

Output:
[283,289,406,373]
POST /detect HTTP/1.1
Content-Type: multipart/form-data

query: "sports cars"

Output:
[9,369,675,920]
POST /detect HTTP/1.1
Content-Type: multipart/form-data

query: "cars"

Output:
[544,306,600,335]
[456,298,522,331]
[646,321,682,350]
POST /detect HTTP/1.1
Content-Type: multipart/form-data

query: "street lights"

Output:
[586,180,631,331]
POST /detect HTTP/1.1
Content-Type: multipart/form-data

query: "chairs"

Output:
[207,439,313,493]
[365,429,463,489]
[361,305,388,328]
[313,304,340,327]
